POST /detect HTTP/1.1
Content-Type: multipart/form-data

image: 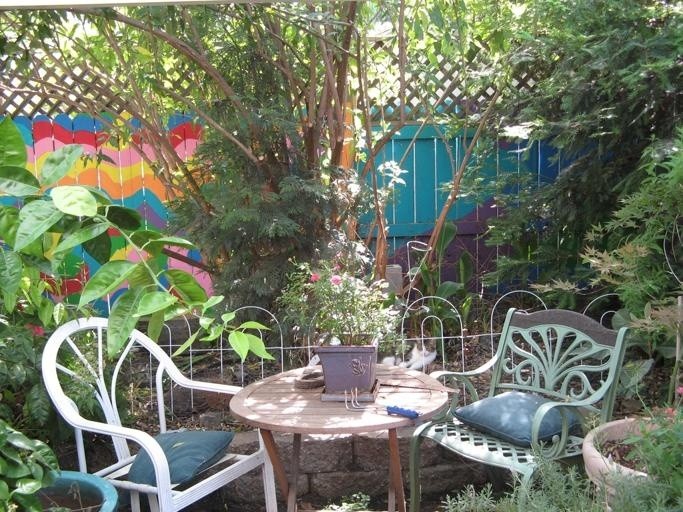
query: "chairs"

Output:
[407,302,630,508]
[39,314,277,512]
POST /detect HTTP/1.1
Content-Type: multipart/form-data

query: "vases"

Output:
[310,333,380,402]
[579,416,662,509]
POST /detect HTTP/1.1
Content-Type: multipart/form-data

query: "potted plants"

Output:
[1,414,119,512]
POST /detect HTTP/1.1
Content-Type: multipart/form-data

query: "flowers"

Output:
[271,253,409,358]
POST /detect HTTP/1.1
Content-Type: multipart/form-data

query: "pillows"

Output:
[446,387,583,450]
[127,428,233,485]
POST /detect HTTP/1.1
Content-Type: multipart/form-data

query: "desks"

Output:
[229,363,448,512]
